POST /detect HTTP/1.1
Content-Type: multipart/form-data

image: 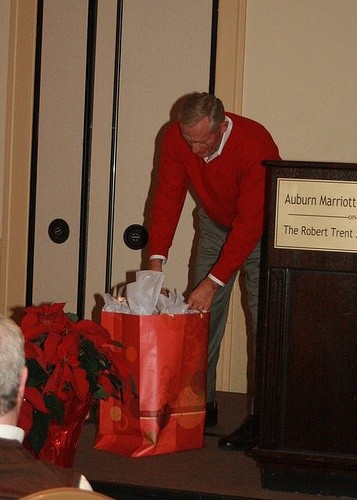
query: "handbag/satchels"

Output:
[95,311,211,458]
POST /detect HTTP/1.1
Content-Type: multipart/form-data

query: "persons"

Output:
[0,316,79,500]
[147,91,282,451]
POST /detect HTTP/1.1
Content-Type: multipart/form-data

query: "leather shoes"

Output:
[205,400,218,426]
[219,416,260,450]
